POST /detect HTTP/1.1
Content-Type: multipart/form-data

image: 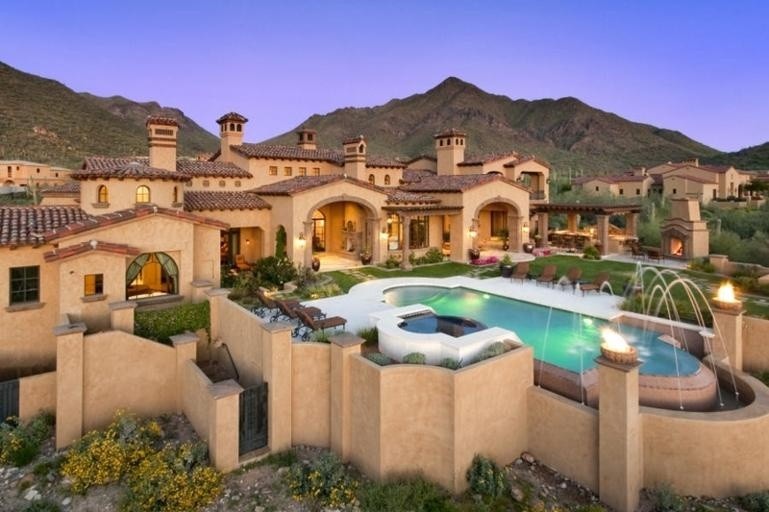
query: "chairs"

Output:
[534,264,610,298]
[647,248,665,264]
[631,242,645,263]
[510,261,530,289]
[249,289,347,342]
